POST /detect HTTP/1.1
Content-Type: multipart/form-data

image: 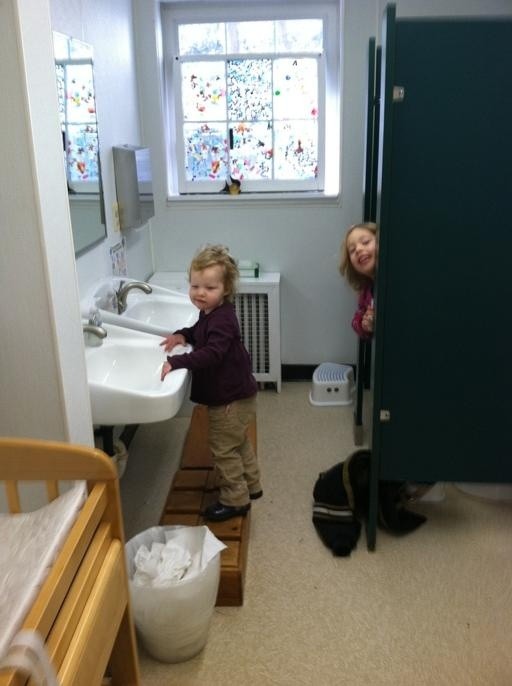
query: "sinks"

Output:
[93,275,200,337]
[82,318,194,424]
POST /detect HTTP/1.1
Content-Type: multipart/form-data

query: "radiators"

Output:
[231,272,282,394]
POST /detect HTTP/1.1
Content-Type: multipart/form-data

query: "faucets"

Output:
[83,324,107,339]
[113,279,152,314]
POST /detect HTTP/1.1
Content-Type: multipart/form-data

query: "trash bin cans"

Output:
[125,525,221,664]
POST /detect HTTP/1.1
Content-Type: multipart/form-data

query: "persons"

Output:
[159,242,264,521]
[338,222,377,339]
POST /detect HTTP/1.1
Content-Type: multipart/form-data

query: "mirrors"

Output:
[50,30,108,260]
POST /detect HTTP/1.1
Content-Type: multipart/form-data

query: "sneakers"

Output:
[206,489,263,522]
